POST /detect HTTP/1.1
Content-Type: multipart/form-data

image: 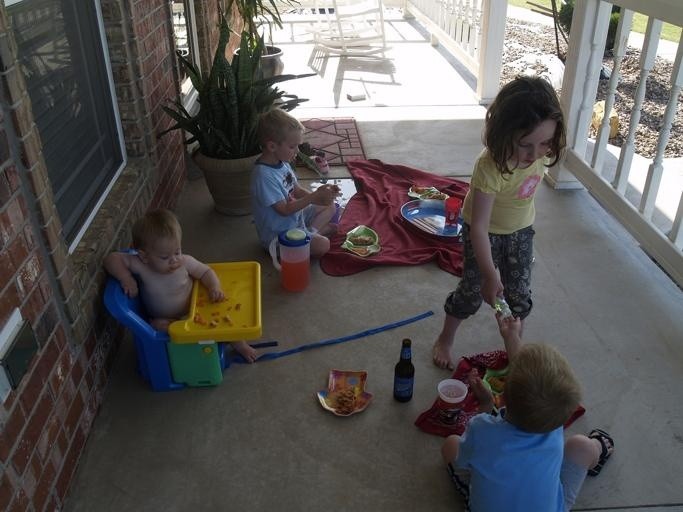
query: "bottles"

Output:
[393,337,415,402]
[266,227,310,292]
[495,296,511,318]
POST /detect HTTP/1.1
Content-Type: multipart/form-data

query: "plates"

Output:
[406,185,450,201]
[315,369,374,417]
[481,366,508,394]
[399,199,466,239]
[339,224,380,257]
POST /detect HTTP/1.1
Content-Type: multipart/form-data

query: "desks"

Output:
[166,258,264,345]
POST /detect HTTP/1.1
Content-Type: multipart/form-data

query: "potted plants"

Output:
[155,0,318,215]
[210,0,303,88]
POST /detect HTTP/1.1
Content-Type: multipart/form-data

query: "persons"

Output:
[104,208,261,364]
[433,77,566,371]
[250,108,340,258]
[441,310,613,512]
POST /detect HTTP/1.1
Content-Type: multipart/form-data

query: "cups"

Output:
[435,379,467,426]
[445,197,462,225]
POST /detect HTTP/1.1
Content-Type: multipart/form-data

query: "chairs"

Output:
[104,249,228,391]
[310,0,392,58]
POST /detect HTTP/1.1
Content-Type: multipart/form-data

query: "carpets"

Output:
[291,116,367,167]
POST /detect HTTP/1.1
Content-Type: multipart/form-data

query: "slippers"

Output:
[587,427,615,478]
